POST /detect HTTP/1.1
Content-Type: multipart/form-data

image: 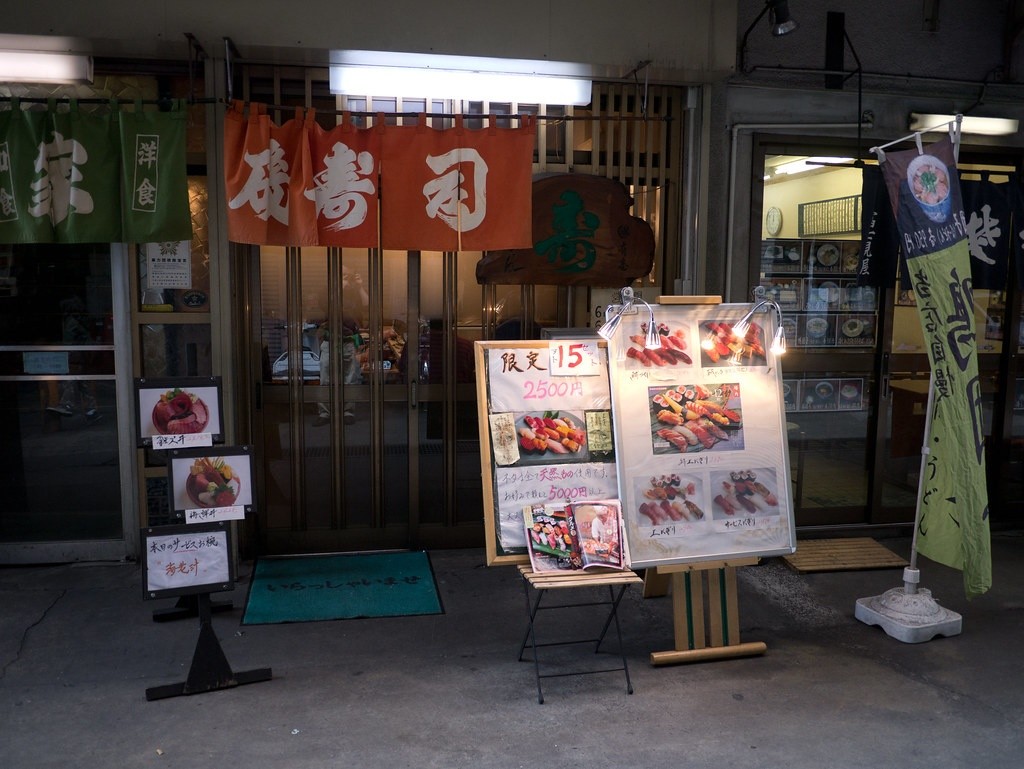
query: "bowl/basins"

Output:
[778,318,796,339]
[151,398,209,435]
[185,473,241,508]
[907,154,952,223]
[783,382,858,403]
[807,243,876,344]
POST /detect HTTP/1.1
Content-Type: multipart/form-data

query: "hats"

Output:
[343,265,350,275]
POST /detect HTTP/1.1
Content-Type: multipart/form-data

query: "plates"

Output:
[780,290,798,304]
[764,246,784,258]
[510,410,591,466]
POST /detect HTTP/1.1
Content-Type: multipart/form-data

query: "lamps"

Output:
[773,155,855,175]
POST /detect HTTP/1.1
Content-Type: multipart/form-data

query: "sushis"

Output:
[627,321,766,365]
[640,385,778,528]
[518,415,582,572]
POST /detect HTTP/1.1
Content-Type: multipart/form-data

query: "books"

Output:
[522,501,632,572]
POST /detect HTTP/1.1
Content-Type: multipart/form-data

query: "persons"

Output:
[591,507,619,559]
[397,312,474,387]
[311,264,369,426]
[44,306,104,426]
[488,290,543,341]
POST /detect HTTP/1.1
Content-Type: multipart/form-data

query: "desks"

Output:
[886,378,931,494]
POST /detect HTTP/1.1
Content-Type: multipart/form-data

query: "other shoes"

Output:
[343,415,354,424]
[312,417,331,427]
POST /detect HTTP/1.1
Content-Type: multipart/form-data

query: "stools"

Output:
[515,562,645,705]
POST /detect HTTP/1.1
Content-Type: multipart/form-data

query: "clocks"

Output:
[765,207,784,236]
